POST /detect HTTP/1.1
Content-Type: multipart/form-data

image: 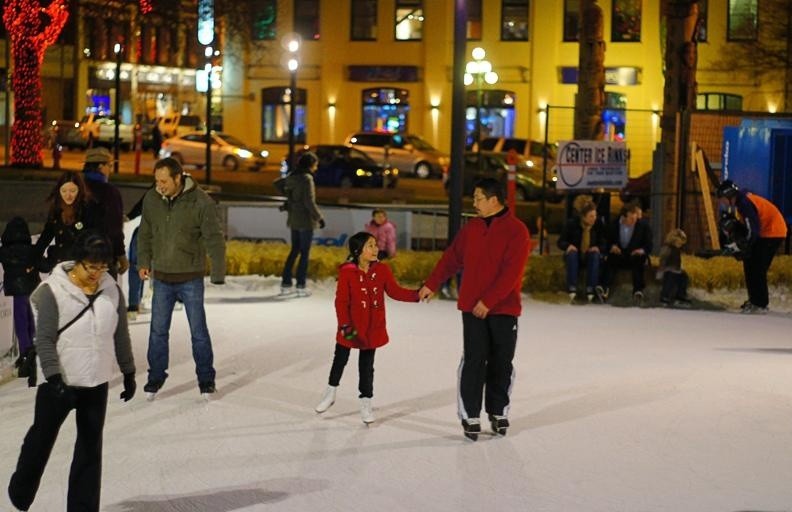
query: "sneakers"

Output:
[458,412,481,433]
[78,147,120,163]
[487,412,512,431]
[124,303,140,312]
[142,370,170,394]
[13,346,33,378]
[739,299,769,314]
[567,286,693,309]
[276,280,312,294]
[194,366,217,395]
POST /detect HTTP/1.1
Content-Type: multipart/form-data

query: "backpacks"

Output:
[716,193,753,260]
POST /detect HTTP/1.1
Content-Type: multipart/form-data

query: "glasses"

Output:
[79,259,109,274]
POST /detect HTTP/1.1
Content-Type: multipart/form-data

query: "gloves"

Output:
[339,325,357,341]
[117,255,131,275]
[46,372,66,397]
[119,374,137,404]
[318,219,326,230]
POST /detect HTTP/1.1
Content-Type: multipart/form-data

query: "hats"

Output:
[296,151,320,168]
[345,231,376,259]
[714,180,739,199]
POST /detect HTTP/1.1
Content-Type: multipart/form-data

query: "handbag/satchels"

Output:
[29,350,40,386]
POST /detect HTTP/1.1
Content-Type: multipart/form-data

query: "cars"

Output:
[619,168,651,209]
[52,111,267,174]
[289,144,399,191]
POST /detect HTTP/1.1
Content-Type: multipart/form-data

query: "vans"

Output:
[344,130,450,180]
[444,134,565,205]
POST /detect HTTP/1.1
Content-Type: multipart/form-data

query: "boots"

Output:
[316,386,339,412]
[359,397,375,423]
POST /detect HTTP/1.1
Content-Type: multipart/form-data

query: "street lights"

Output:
[107,43,126,172]
[194,43,225,184]
[463,46,498,164]
[282,39,300,163]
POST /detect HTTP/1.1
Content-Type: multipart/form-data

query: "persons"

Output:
[151,123,165,157]
[365,207,398,258]
[317,233,427,426]
[419,176,532,437]
[273,154,328,296]
[557,179,788,315]
